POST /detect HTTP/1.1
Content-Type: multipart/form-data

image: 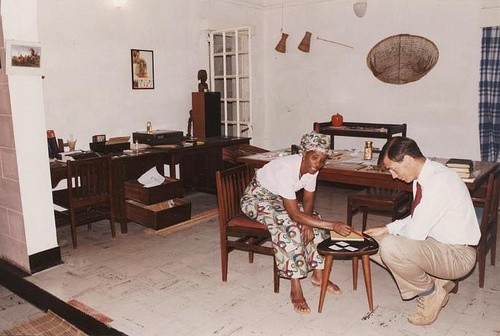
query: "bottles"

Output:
[147,123,152,133]
[66,134,77,151]
[48,129,58,157]
[364,141,372,160]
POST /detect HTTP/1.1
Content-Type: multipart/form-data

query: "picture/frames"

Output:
[131,49,154,89]
[5,39,46,76]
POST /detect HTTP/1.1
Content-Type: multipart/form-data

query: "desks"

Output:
[237,148,500,193]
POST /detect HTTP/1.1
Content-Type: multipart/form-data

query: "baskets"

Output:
[366,32,440,84]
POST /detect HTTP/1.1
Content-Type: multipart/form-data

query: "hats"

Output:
[299,131,338,154]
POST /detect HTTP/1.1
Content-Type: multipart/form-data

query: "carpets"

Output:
[0,309,87,336]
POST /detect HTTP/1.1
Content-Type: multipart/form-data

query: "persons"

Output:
[362,137,482,325]
[238,130,353,315]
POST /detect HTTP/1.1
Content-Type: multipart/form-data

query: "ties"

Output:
[410,181,422,218]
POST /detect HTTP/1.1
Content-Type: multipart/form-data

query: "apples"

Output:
[331,113,343,127]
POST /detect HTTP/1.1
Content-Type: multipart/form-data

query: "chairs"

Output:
[474,169,500,287]
[53,155,116,249]
[215,162,279,293]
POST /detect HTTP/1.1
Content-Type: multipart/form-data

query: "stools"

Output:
[347,187,413,233]
[317,233,379,312]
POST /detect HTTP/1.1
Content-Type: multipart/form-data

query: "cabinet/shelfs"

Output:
[50,151,108,228]
[179,137,251,196]
[314,122,406,150]
[109,149,191,231]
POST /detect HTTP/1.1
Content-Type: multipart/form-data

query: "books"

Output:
[445,157,474,181]
[330,229,365,242]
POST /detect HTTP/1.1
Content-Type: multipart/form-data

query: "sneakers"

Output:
[407,283,449,326]
[434,275,457,295]
